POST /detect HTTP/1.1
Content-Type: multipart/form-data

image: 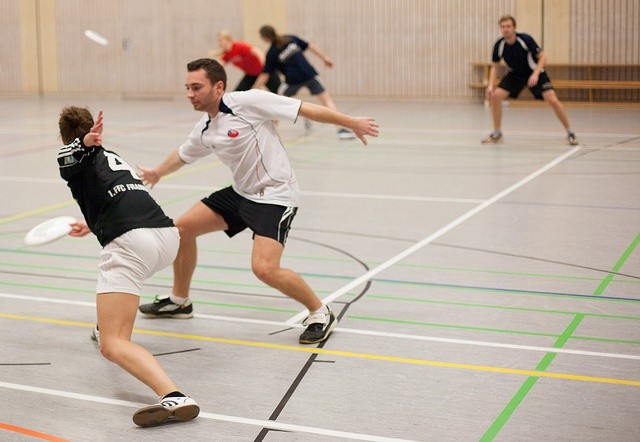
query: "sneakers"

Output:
[300,306,337,343]
[132,395,199,425]
[567,132,578,145]
[304,119,312,130]
[338,128,357,139]
[138,295,192,318]
[90,327,100,343]
[482,133,504,145]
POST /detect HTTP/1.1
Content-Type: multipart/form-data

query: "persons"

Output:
[136,58,380,343]
[482,15,576,146]
[215,31,266,91]
[58,107,200,426]
[260,25,359,138]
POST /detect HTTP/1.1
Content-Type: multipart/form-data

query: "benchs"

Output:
[469,63,640,107]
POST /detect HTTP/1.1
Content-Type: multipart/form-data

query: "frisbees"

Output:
[24,216,76,246]
